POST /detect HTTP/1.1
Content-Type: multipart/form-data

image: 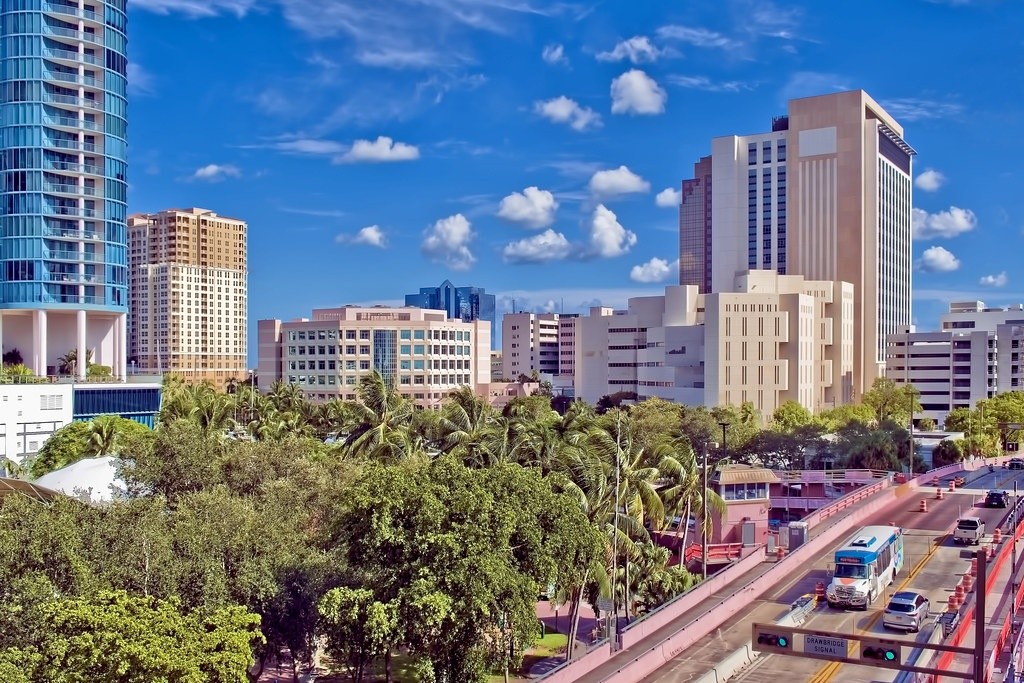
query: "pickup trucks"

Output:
[952,516,987,545]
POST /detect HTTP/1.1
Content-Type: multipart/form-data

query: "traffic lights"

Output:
[857,640,900,664]
[757,629,791,650]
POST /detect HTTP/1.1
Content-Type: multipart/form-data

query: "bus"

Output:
[825,525,904,612]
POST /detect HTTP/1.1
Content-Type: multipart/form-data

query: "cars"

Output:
[1008,458,1024,470]
[882,591,930,632]
[984,490,1010,508]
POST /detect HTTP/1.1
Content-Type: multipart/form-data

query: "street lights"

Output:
[247,369,255,419]
[903,391,921,480]
